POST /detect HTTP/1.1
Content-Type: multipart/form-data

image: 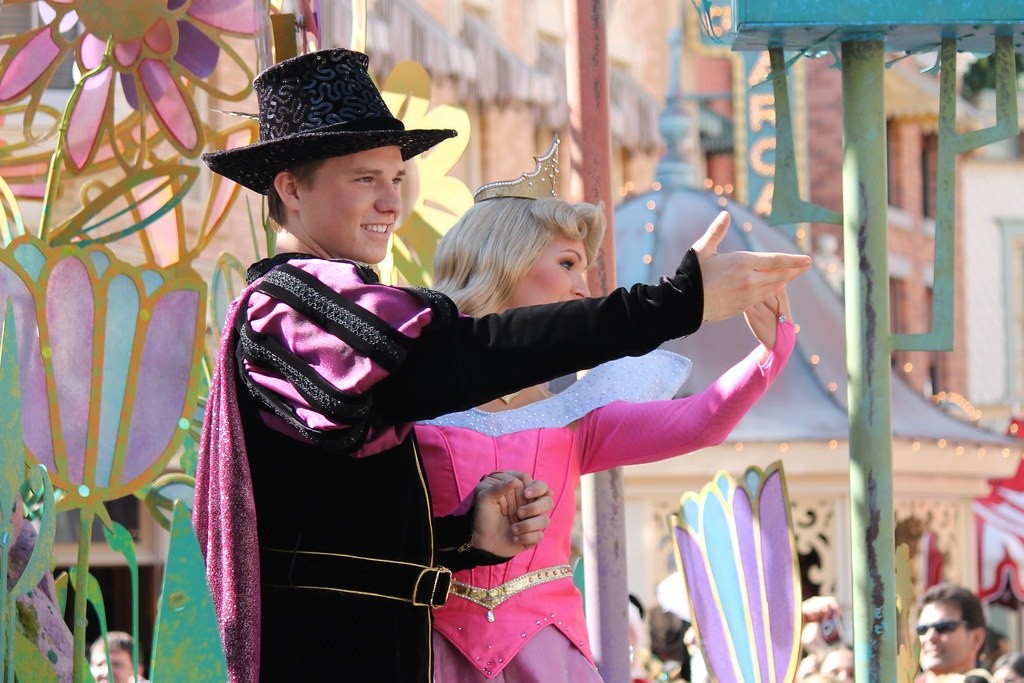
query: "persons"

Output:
[411,137,796,683]
[192,47,811,683]
[914,585,1007,683]
[90,631,143,683]
[816,643,855,683]
[992,652,1024,683]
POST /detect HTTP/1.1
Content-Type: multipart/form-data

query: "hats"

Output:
[202,48,458,196]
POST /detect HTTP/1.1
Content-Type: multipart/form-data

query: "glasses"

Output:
[916,621,972,636]
